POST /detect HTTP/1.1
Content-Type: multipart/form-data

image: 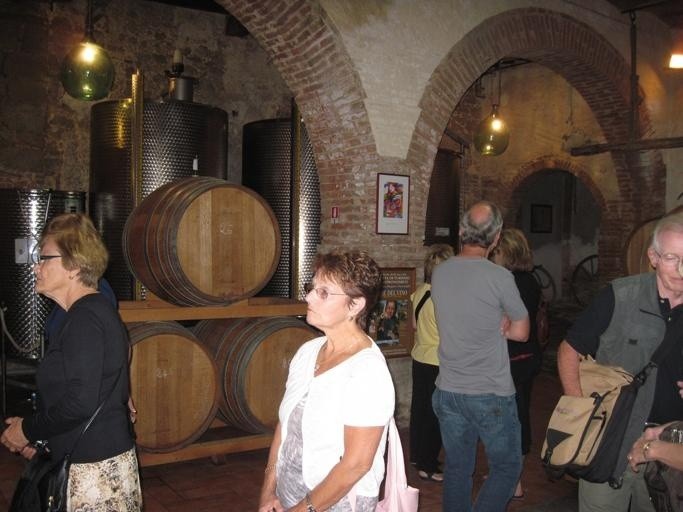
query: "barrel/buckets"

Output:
[192,315,325,435]
[120,175,283,307]
[125,320,222,454]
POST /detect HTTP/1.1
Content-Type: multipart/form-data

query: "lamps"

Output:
[474,66,510,158]
[59,1,116,101]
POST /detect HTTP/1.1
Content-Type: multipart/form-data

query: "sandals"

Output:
[416,467,443,482]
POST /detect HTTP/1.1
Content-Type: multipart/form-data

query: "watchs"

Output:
[642,438,655,460]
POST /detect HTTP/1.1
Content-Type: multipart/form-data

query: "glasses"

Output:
[652,248,682,266]
[35,253,64,263]
[303,282,348,299]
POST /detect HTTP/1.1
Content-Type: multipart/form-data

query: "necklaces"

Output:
[313,337,363,371]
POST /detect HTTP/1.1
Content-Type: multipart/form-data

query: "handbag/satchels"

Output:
[644,422,683,512]
[541,310,681,482]
[375,416,418,512]
[8,324,131,511]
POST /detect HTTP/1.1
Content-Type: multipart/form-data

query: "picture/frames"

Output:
[365,266,417,358]
[376,172,410,235]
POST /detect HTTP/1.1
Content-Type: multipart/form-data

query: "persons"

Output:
[375,300,400,340]
[409,244,455,482]
[675,379,683,399]
[556,212,683,512]
[0,225,144,512]
[626,420,683,473]
[430,200,531,512]
[383,182,403,219]
[258,250,396,512]
[41,214,137,425]
[493,228,542,502]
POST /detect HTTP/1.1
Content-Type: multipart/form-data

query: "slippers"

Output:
[512,496,522,500]
